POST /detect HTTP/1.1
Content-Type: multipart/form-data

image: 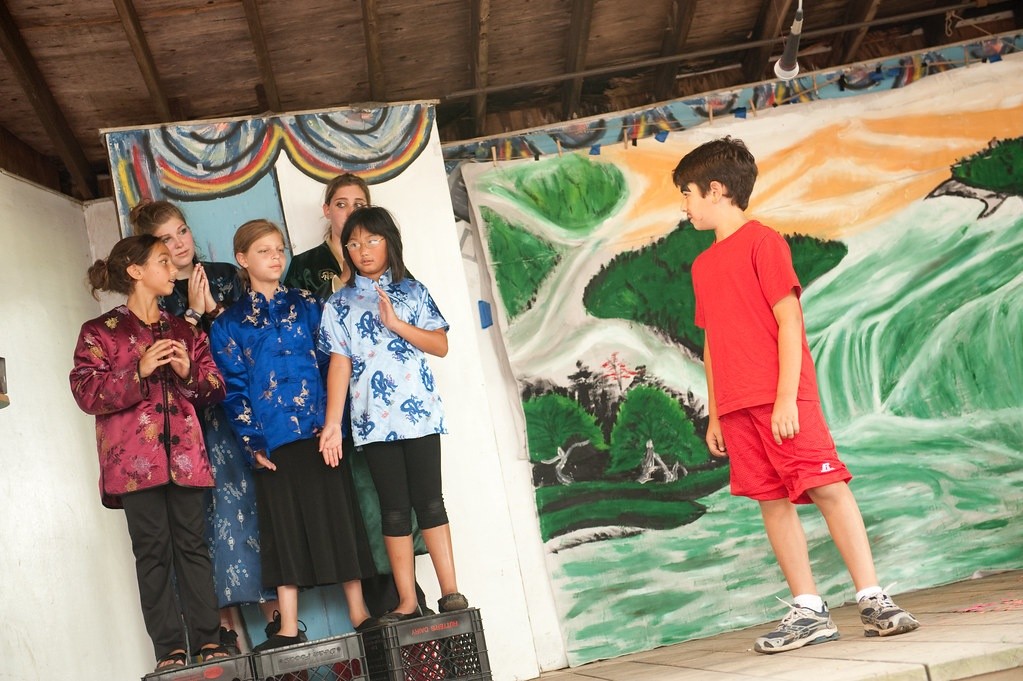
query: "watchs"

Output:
[184,308,201,321]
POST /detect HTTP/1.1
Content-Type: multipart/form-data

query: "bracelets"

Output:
[207,302,222,319]
[195,311,202,316]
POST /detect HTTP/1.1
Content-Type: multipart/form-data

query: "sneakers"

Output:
[754,596,841,654]
[857,582,921,637]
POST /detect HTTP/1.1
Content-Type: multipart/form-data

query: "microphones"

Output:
[774,10,804,81]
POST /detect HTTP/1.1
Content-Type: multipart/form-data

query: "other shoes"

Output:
[379,603,423,624]
[352,615,384,633]
[252,627,301,653]
[438,593,468,612]
[265,610,308,642]
[219,626,241,655]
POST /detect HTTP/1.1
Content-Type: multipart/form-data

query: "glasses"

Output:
[345,237,385,251]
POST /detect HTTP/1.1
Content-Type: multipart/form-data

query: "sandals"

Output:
[199,643,231,662]
[154,650,187,672]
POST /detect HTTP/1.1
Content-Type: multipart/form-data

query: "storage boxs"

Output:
[140,608,494,681]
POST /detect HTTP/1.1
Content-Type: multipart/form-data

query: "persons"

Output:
[128,200,281,656]
[283,176,436,615]
[206,219,389,650]
[673,133,920,654]
[69,235,232,672]
[320,208,468,625]
